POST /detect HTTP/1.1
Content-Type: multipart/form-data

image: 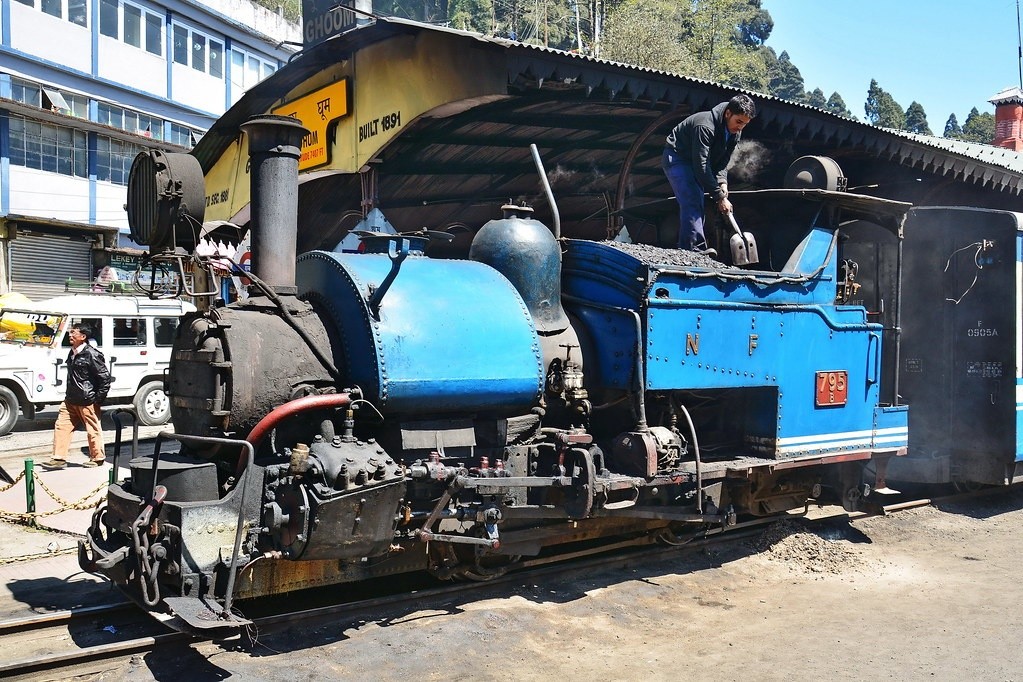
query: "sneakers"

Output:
[83,460,104,468]
[42,460,65,470]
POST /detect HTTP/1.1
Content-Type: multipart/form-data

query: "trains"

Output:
[70,101,1022,649]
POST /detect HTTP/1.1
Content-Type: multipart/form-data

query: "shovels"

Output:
[728,212,759,265]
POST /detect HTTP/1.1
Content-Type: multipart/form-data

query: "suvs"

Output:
[0,277,200,437]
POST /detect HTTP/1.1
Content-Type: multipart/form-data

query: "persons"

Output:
[41,324,112,471]
[659,94,757,258]
